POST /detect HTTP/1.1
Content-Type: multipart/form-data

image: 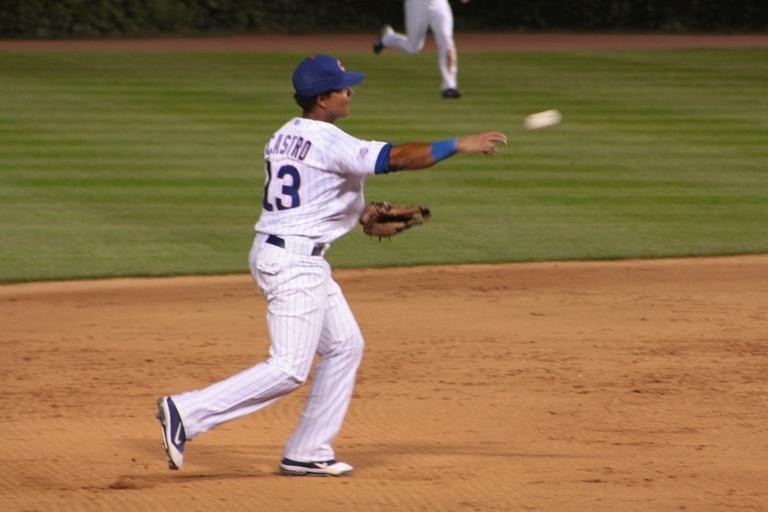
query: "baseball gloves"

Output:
[364,204,430,236]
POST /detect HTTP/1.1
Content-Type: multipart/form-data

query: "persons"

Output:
[156,54,507,478]
[372,0,464,99]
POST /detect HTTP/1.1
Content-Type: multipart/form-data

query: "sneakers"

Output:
[441,88,461,100]
[371,24,391,56]
[277,455,358,479]
[156,393,187,473]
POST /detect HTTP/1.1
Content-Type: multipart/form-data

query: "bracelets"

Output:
[430,136,460,162]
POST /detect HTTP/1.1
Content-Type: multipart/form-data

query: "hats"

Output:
[288,53,367,99]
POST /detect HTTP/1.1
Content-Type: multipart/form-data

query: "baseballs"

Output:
[525,109,561,130]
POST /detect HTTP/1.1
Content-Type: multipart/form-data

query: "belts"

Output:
[254,229,331,258]
